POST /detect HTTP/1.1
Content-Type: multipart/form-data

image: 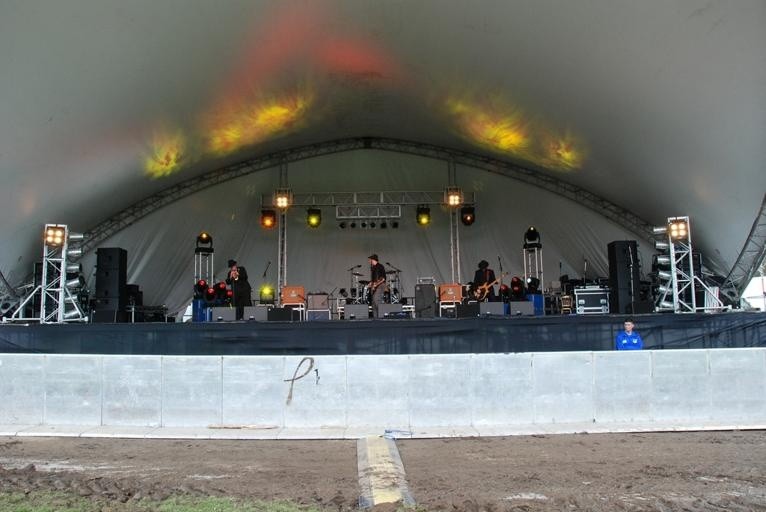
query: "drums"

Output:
[363,283,388,305]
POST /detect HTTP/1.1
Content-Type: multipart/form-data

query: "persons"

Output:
[225,259,252,318]
[614,317,642,350]
[366,254,385,319]
[472,259,500,301]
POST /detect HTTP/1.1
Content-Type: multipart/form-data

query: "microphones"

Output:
[386,263,390,265]
[321,302,325,305]
[560,260,562,269]
[268,261,272,265]
[497,254,501,260]
[357,264,361,267]
[584,259,587,272]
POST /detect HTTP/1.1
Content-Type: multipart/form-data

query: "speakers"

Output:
[438,283,462,302]
[607,241,640,314]
[281,286,304,304]
[344,304,402,320]
[212,306,268,322]
[307,294,329,310]
[95,248,128,323]
[480,302,504,316]
[506,302,535,316]
[415,284,436,318]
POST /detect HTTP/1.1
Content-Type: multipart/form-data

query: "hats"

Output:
[228,259,237,267]
[478,260,488,269]
[367,254,379,262]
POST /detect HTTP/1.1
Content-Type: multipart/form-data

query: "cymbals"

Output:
[357,280,371,284]
[352,273,365,276]
[386,270,397,274]
[388,280,396,282]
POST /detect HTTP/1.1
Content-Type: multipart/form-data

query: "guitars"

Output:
[474,272,511,300]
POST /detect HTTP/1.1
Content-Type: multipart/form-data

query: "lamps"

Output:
[337,221,401,232]
[522,225,543,249]
[44,223,84,321]
[194,280,232,305]
[652,215,697,315]
[194,233,215,257]
[259,186,323,230]
[415,185,476,227]
